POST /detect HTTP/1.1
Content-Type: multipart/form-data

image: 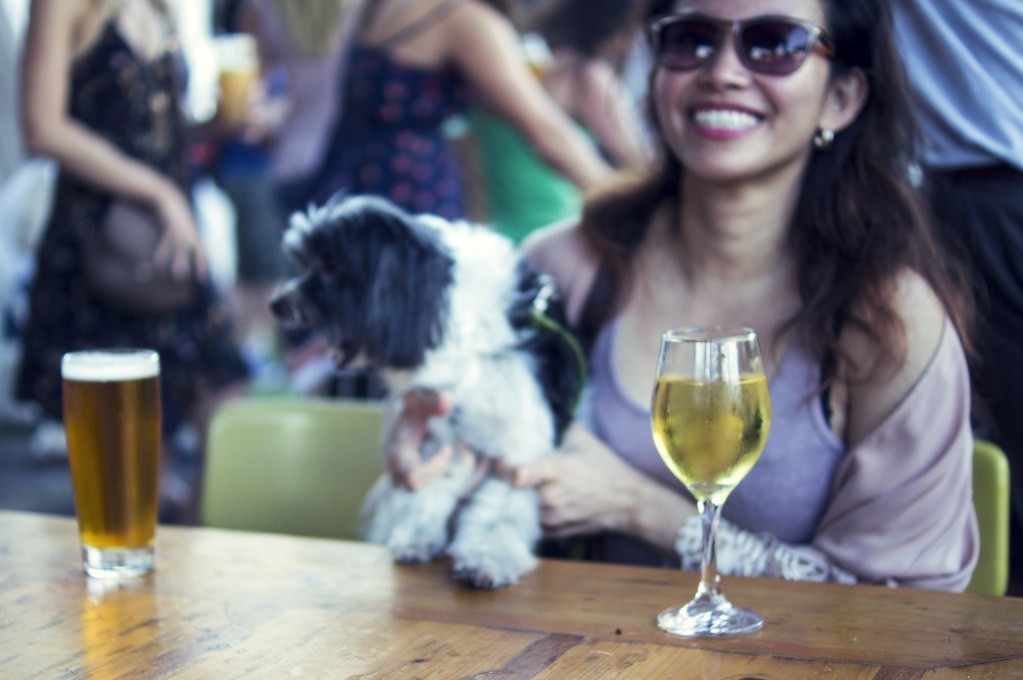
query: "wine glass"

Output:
[649,323,770,637]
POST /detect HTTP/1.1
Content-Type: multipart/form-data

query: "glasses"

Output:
[646,13,834,76]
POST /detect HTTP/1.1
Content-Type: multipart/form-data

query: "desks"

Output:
[0,508,1023,680]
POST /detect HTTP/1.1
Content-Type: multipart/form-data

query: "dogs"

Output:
[270,195,585,590]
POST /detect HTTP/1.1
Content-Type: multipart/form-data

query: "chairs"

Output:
[964,436,1013,597]
[200,396,385,542]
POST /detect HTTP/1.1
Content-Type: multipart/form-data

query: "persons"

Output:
[382,0,979,594]
[883,0,1023,598]
[0,0,656,514]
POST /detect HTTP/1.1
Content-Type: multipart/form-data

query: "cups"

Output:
[62,350,162,583]
